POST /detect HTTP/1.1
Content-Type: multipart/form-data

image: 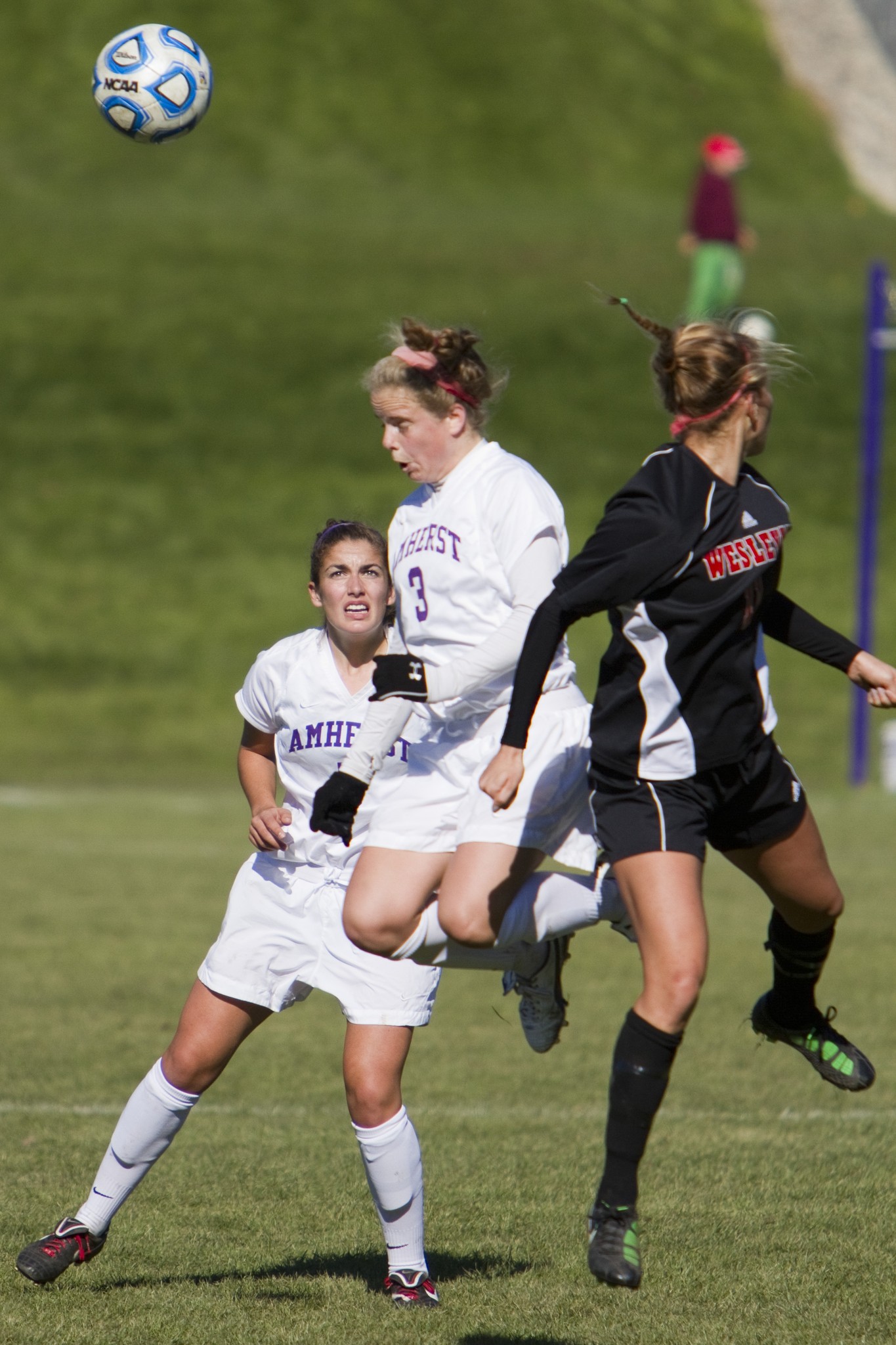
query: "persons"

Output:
[677,134,757,327]
[12,514,449,1309]
[479,293,896,1287]
[308,317,636,1055]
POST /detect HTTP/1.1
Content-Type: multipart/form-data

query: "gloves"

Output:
[368,654,428,703]
[309,771,368,847]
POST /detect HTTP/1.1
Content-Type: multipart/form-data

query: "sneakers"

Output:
[502,930,576,1054]
[384,1269,440,1310]
[751,988,877,1093]
[594,851,638,943]
[15,1216,109,1287]
[586,1195,642,1292]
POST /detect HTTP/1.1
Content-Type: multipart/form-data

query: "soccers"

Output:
[90,23,214,145]
